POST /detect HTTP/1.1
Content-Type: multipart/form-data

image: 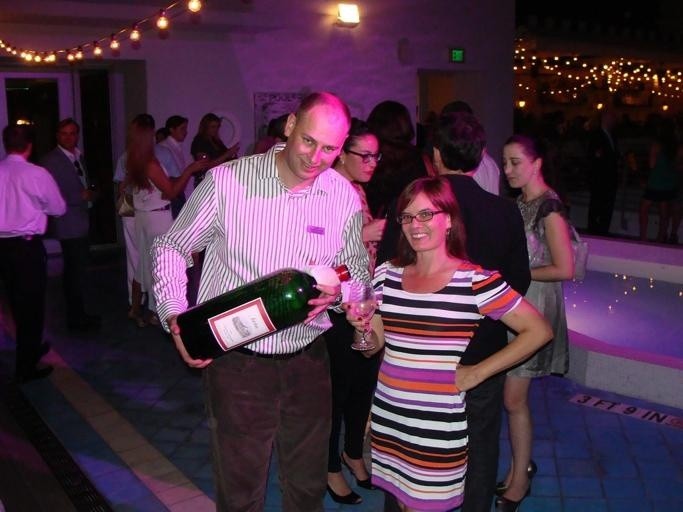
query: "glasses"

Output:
[346,148,382,163]
[74,160,83,176]
[397,209,444,224]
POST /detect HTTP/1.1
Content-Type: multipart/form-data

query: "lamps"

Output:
[336,4,360,29]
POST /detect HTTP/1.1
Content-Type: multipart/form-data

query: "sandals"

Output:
[340,452,376,491]
[326,477,363,505]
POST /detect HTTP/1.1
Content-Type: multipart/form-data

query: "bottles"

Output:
[172,265,352,361]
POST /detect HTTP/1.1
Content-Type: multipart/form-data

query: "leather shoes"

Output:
[493,458,538,510]
[128,311,160,328]
[17,340,55,383]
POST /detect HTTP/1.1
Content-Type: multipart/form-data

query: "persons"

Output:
[377,115,531,512]
[2,123,67,383]
[343,177,555,511]
[49,117,103,325]
[327,99,427,505]
[149,91,369,512]
[114,112,290,328]
[448,99,575,510]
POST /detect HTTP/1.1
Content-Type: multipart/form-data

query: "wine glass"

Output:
[345,279,379,351]
[200,153,208,169]
[86,184,97,202]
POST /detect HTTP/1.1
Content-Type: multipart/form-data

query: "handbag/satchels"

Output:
[525,231,588,281]
[117,193,135,218]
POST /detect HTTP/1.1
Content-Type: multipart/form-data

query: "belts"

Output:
[236,335,320,360]
